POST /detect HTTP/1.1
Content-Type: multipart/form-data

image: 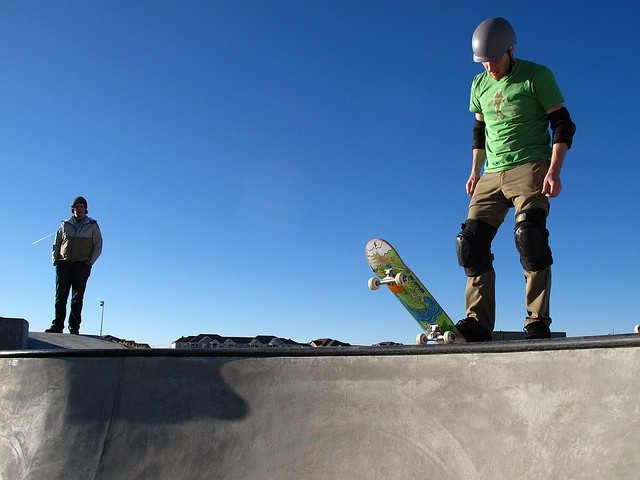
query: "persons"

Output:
[45,197,103,335]
[454,17,576,342]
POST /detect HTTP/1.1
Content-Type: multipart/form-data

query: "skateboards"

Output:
[365,238,466,344]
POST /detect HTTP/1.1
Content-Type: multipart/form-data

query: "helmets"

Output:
[471,17,517,63]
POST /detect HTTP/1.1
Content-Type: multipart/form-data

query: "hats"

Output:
[71,197,87,209]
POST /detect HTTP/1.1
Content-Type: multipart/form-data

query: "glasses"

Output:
[74,205,85,208]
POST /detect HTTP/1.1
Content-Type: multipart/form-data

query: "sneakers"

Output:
[45,328,64,333]
[523,321,551,339]
[455,317,492,341]
[70,329,79,335]
